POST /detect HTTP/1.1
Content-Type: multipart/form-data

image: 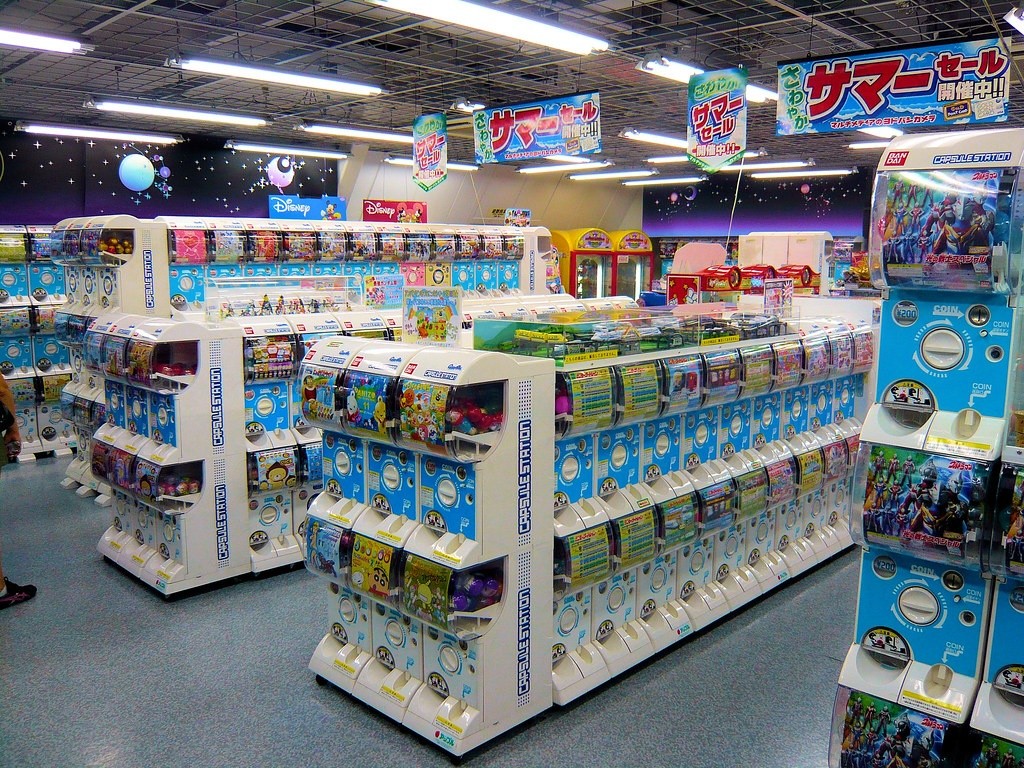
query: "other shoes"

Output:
[0,576,37,612]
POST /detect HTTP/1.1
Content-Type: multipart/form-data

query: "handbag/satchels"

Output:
[0,400,15,431]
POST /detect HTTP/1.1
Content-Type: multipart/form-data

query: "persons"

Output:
[0,372,37,608]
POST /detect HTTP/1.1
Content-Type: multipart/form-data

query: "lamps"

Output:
[618,126,689,149]
[744,165,860,179]
[455,96,492,113]
[543,154,592,163]
[0,26,98,55]
[568,166,660,181]
[164,50,396,98]
[512,159,617,175]
[640,146,769,164]
[855,126,905,139]
[14,120,186,147]
[637,50,778,103]
[223,139,356,160]
[82,94,277,128]
[370,0,625,57]
[718,156,816,171]
[1003,6,1024,35]
[293,122,414,143]
[621,174,709,186]
[842,139,890,149]
[384,154,484,172]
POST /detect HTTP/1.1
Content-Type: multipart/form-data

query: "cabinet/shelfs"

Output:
[739,230,837,296]
[834,235,867,287]
[56,224,524,512]
[734,294,884,421]
[78,295,643,602]
[520,227,553,295]
[0,224,77,463]
[825,126,1024,768]
[279,314,876,768]
